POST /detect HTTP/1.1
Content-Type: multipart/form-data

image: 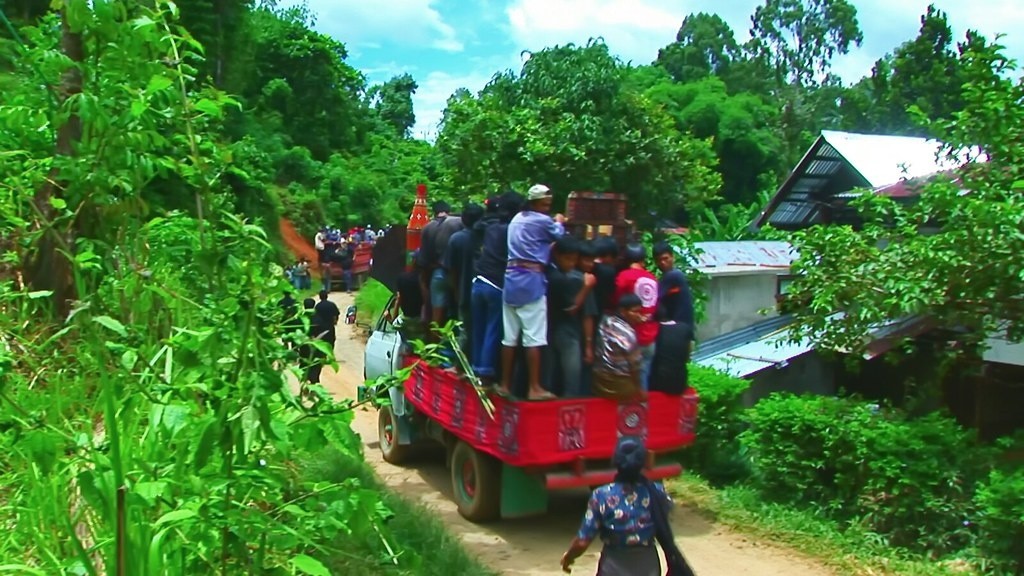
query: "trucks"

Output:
[316,240,372,295]
[355,293,702,525]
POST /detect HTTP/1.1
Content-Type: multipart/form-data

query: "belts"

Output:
[506,260,545,272]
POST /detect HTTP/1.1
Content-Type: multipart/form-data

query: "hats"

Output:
[527,183,553,200]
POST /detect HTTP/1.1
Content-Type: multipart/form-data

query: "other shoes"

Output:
[493,382,513,397]
[474,375,497,390]
[527,389,557,401]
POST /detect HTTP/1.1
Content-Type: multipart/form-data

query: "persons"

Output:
[559,438,678,576]
[273,288,339,385]
[315,224,385,294]
[382,185,698,399]
[285,259,311,291]
